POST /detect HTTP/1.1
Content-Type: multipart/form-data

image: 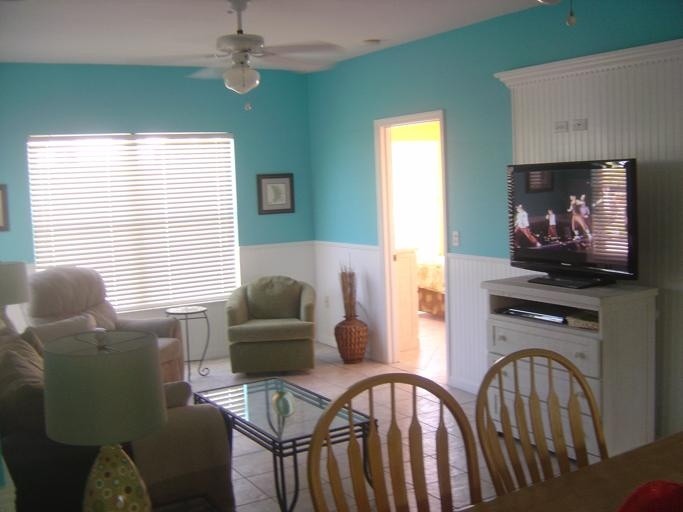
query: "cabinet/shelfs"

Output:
[479,273,659,466]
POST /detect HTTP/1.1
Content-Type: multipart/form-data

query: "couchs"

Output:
[225,274,317,373]
[22,263,185,384]
[0,336,236,512]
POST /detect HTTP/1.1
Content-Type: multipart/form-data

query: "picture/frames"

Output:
[0,184,11,238]
[256,172,296,216]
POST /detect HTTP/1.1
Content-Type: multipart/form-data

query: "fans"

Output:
[173,1,345,96]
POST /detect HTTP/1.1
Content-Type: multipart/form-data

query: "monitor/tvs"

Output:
[508,158,639,288]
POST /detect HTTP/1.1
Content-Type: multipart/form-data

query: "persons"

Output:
[513,202,542,249]
[576,190,590,233]
[545,207,561,244]
[566,193,590,241]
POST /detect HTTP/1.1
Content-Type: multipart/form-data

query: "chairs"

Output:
[475,348,609,499]
[305,372,482,511]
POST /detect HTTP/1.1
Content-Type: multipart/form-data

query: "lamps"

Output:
[0,260,29,309]
[41,329,170,510]
[215,34,264,96]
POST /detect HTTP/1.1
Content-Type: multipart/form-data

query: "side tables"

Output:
[165,304,211,382]
